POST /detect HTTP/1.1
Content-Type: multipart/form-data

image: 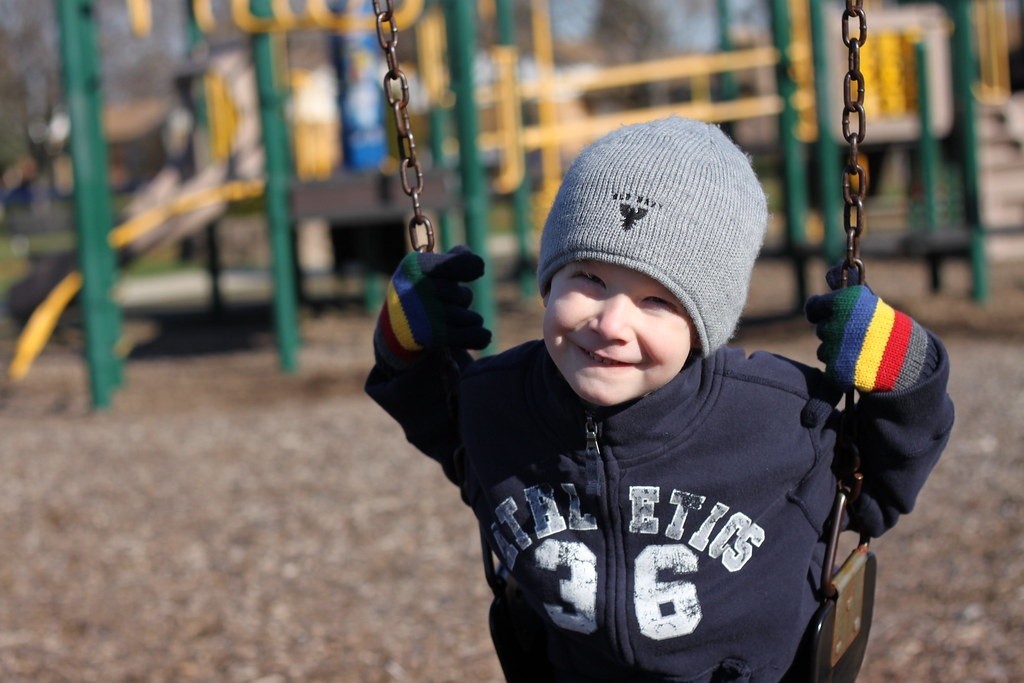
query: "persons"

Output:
[362,115,956,683]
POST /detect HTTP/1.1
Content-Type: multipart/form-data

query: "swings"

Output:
[369,0,879,683]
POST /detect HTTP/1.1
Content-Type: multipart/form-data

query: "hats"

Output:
[538,115,769,358]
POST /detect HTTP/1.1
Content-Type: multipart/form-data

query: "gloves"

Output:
[373,243,493,364]
[806,267,940,394]
